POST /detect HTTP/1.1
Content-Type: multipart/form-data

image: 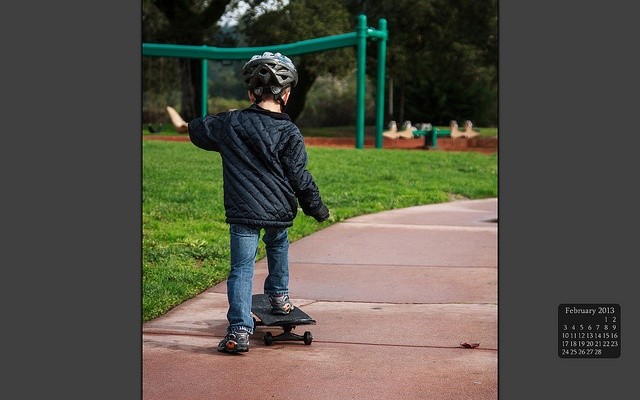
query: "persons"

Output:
[166,51,329,353]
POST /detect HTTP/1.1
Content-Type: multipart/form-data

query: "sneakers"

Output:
[218,328,251,354]
[269,292,295,316]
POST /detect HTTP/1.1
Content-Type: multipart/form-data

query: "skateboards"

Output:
[251,294,317,345]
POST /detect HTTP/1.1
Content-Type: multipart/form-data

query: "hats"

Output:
[242,50,299,94]
